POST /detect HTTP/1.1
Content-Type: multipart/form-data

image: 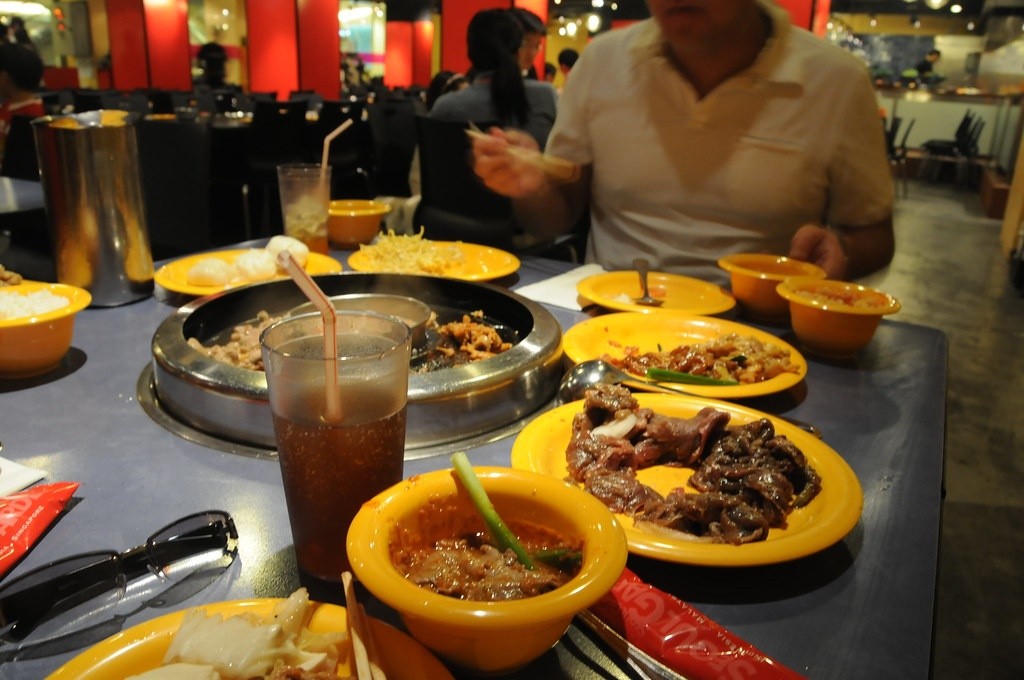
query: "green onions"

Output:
[647,368,737,385]
[450,451,531,566]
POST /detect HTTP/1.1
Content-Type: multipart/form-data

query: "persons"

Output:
[469,0,896,310]
[0,18,54,243]
[899,48,942,103]
[413,6,586,254]
[189,41,244,100]
[339,50,370,94]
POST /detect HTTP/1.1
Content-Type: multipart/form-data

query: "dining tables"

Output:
[0,228,950,678]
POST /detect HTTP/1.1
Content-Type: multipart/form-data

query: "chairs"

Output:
[0,70,593,280]
[886,108,987,195]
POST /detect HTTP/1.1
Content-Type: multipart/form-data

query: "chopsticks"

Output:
[464,128,575,181]
[339,567,387,680]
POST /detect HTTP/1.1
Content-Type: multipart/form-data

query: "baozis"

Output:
[187,236,308,287]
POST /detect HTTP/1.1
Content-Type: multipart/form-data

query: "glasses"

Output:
[520,39,541,51]
[0,510,237,647]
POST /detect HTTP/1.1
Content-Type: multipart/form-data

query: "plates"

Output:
[562,312,808,399]
[153,248,344,297]
[44,597,458,680]
[347,240,521,282]
[511,390,864,567]
[576,269,736,317]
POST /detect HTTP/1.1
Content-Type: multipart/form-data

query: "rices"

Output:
[0,288,69,320]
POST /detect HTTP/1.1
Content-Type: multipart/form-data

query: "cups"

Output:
[276,163,332,256]
[258,309,414,589]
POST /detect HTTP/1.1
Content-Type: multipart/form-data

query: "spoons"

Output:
[632,258,664,307]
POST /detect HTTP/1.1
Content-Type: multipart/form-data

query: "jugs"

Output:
[31,109,153,309]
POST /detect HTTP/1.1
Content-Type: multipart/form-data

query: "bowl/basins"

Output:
[0,279,92,379]
[717,252,828,320]
[777,279,901,361]
[345,466,629,678]
[328,199,392,250]
